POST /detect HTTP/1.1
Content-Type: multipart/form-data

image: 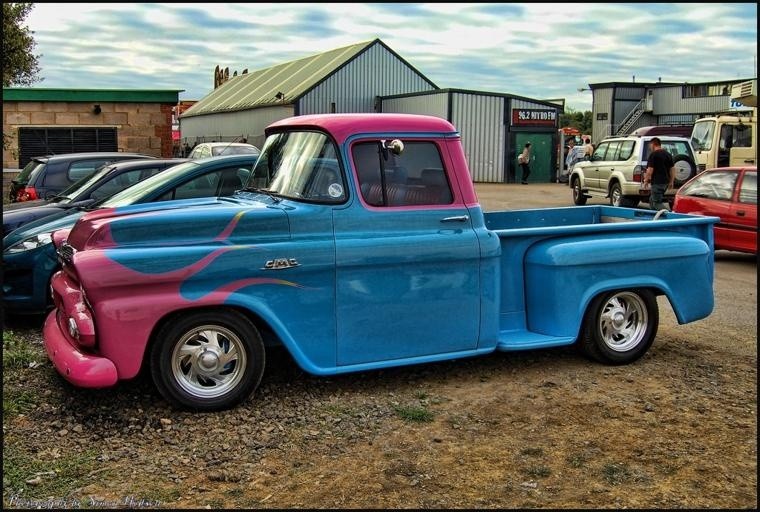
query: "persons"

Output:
[565,140,577,186]
[521,143,532,184]
[584,138,593,157]
[644,137,676,210]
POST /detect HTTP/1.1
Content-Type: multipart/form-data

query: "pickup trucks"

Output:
[42,113,720,411]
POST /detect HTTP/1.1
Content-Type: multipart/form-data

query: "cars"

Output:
[673,165,758,254]
[2,142,341,321]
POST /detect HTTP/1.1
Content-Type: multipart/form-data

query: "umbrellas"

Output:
[521,180,528,184]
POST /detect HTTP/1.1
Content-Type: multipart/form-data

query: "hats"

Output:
[673,165,758,254]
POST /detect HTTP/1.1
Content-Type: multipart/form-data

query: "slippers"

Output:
[518,154,524,164]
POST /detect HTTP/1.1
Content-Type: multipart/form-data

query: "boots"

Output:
[569,134,697,208]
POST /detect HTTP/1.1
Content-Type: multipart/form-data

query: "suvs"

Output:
[569,134,697,208]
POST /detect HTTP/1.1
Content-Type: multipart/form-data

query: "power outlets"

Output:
[42,113,720,411]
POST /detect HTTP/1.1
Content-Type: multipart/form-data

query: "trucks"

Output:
[691,115,756,171]
[630,125,694,138]
